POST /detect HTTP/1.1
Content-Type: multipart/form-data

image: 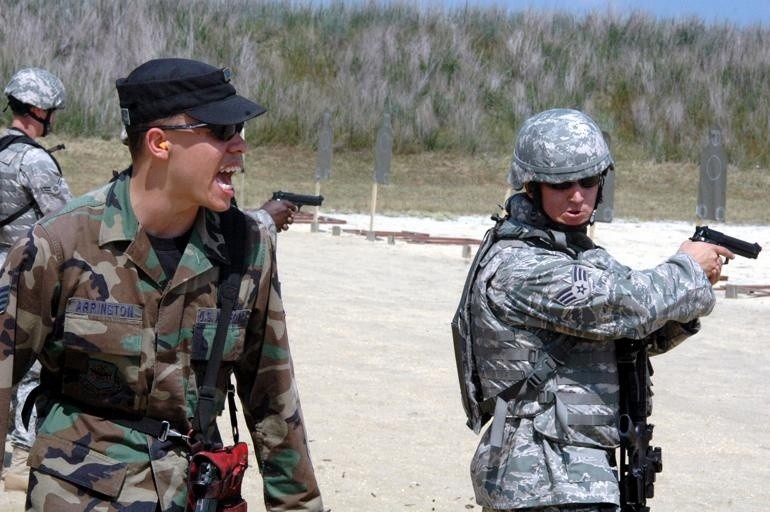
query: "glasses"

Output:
[134,121,244,142]
[546,173,602,190]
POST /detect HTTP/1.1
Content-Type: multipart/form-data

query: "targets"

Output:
[695,129,728,223]
[372,113,394,185]
[314,112,333,182]
[593,130,615,223]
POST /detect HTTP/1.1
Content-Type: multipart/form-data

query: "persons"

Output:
[455,108,734,512]
[0,57,328,512]
[0,68,77,272]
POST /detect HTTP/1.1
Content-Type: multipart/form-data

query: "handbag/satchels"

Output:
[185,441,248,512]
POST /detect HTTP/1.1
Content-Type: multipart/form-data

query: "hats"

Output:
[116,58,267,125]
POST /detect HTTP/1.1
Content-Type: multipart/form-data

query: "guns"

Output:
[269,190,324,227]
[614,337,662,512]
[688,225,761,266]
[190,458,221,511]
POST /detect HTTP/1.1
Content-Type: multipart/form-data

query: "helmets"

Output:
[4,67,66,109]
[507,108,615,191]
